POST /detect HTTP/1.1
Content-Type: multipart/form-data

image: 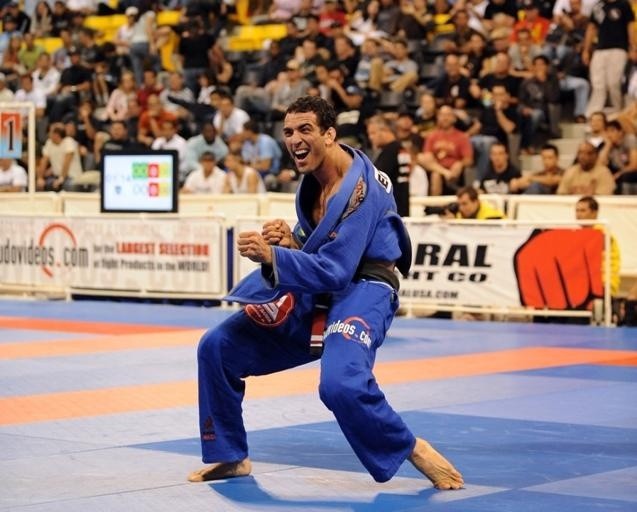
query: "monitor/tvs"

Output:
[99,148,179,212]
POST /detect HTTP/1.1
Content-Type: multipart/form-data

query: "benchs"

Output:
[517,106,620,175]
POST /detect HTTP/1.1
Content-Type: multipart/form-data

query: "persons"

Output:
[187,96,464,491]
[0,0,637,220]
[427,186,506,322]
[577,195,627,323]
